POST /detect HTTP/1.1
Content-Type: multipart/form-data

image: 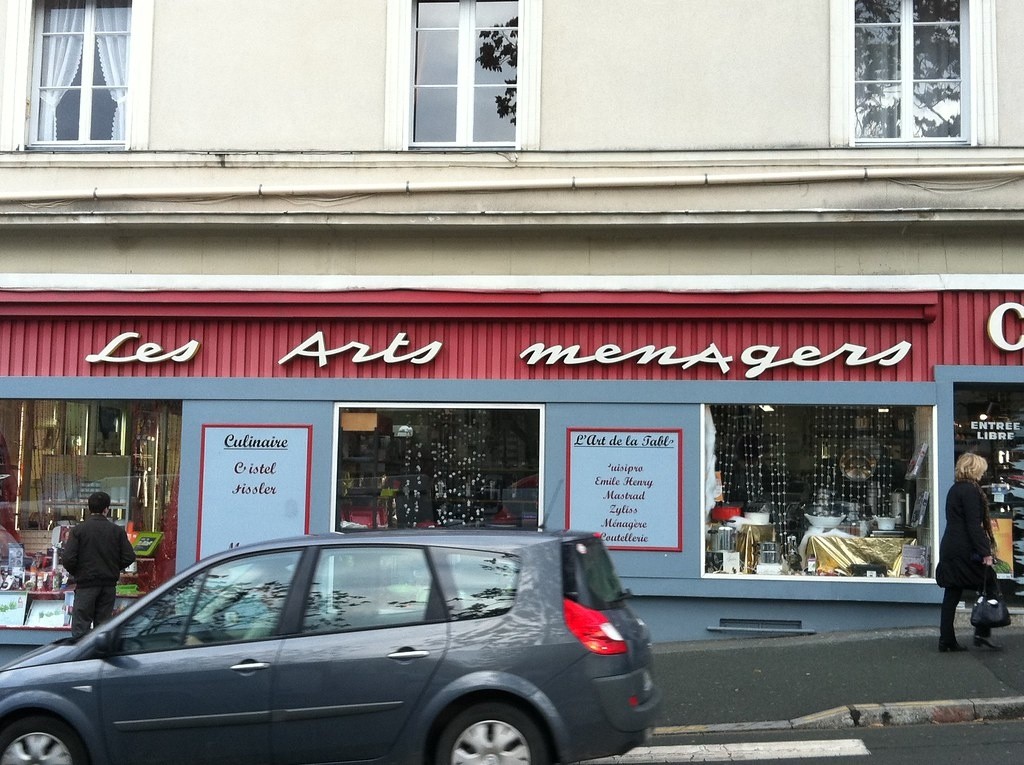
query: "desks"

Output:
[708,522,777,574]
[804,534,915,576]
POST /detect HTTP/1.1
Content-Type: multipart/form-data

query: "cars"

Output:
[47,475,176,525]
[726,490,865,540]
[0,530,655,765]
[336,474,538,528]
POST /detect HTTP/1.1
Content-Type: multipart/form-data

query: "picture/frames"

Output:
[132,531,164,558]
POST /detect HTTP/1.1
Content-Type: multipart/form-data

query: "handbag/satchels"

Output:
[971,565,1011,628]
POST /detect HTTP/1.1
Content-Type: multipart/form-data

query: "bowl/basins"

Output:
[804,513,846,528]
[877,517,896,530]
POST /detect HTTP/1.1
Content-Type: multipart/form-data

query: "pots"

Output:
[708,520,736,551]
[757,542,777,563]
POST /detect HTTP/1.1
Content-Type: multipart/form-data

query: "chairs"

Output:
[333,598,378,630]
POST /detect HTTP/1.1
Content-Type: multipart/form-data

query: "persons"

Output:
[62,491,136,636]
[936,453,1003,651]
[185,563,287,647]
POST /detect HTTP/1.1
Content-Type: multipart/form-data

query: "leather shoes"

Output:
[939,635,966,652]
[974,635,1003,649]
